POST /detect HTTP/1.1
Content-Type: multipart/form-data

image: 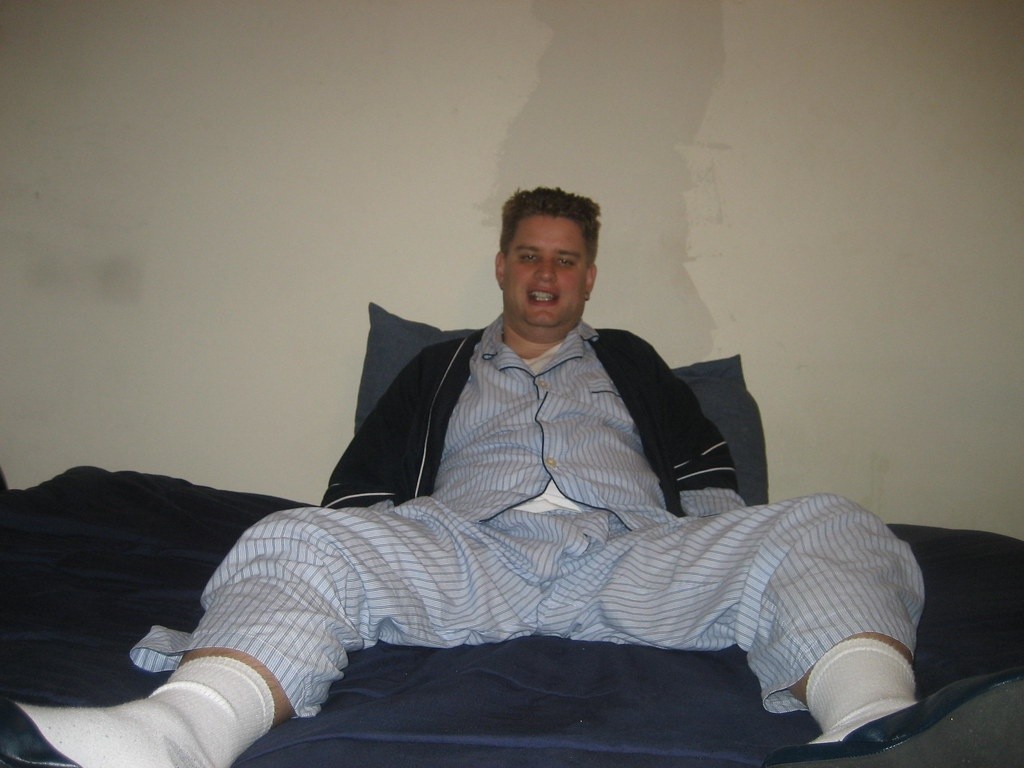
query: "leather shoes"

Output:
[761,668,1024,768]
[0,697,83,768]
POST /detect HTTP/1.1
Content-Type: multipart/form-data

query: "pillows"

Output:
[354,303,770,507]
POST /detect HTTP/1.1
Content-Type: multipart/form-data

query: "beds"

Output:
[0,465,1024,767]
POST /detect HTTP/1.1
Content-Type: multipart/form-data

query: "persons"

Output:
[0,185,1024,768]
